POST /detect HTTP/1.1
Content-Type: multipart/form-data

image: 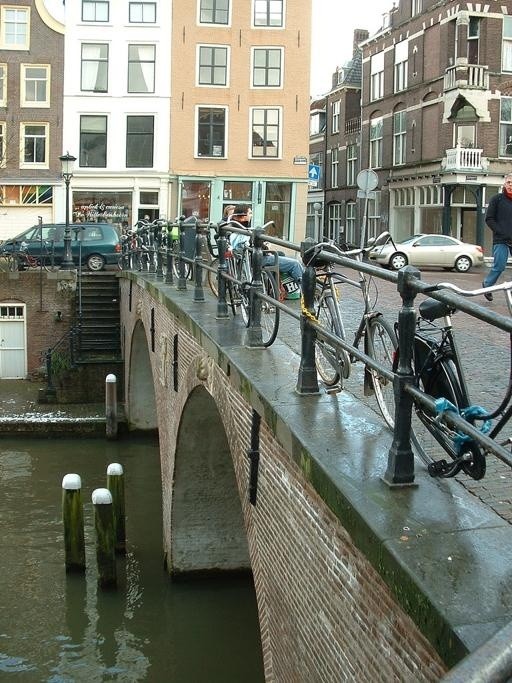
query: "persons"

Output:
[479,173,512,301]
[191,208,200,217]
[221,204,235,221]
[231,202,304,282]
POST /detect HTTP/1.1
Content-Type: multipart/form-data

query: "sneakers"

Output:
[482,280,493,301]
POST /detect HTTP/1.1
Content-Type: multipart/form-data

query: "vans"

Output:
[0,217,123,271]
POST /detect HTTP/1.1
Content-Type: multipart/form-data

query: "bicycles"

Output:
[8,236,62,274]
[391,269,512,485]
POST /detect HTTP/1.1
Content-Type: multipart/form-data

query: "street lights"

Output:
[57,149,80,269]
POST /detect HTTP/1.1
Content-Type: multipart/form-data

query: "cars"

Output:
[367,231,487,274]
